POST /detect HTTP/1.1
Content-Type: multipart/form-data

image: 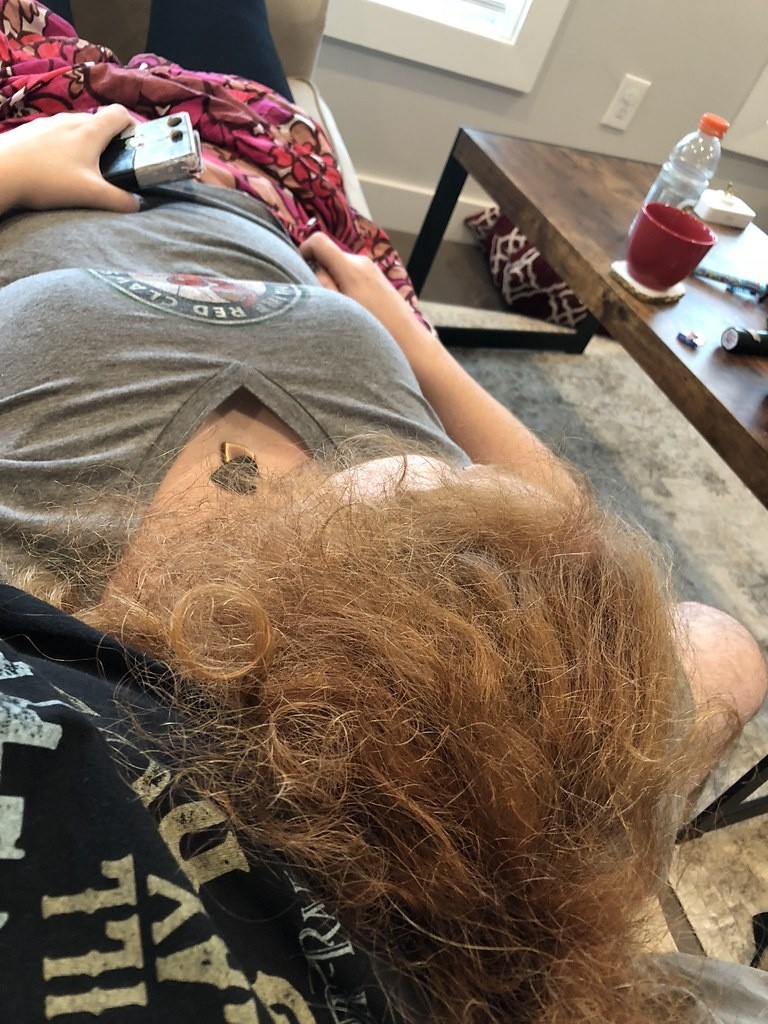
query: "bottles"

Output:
[627,109,729,241]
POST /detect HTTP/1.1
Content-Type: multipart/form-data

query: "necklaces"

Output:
[209,436,260,501]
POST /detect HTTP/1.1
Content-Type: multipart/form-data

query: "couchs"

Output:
[66,0,365,231]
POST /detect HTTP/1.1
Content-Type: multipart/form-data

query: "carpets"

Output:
[414,300,768,970]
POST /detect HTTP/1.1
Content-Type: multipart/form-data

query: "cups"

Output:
[626,202,719,293]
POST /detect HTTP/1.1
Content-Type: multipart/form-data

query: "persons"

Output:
[0,579,768,1024]
[0,0,719,1024]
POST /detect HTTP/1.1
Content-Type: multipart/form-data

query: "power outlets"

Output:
[600,74,652,131]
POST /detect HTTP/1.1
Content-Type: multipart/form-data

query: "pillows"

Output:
[458,205,596,327]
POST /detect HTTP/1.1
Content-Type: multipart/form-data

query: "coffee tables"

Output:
[408,128,766,613]
